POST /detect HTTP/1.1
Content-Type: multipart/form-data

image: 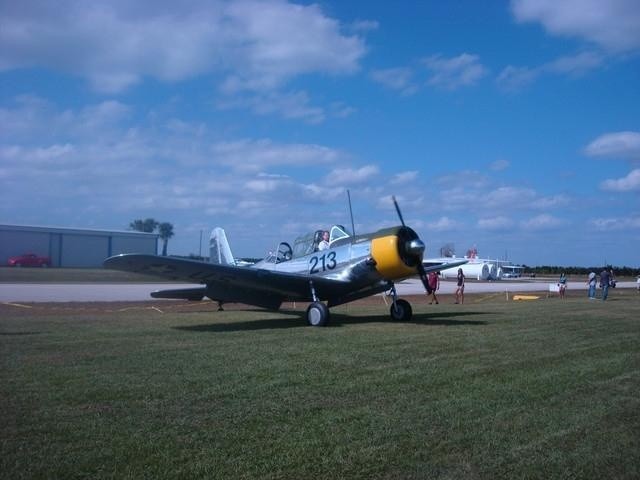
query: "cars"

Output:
[502,271,522,278]
[9,252,52,268]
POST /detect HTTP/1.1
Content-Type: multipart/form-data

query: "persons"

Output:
[559,272,568,300]
[454,268,465,304]
[588,269,597,300]
[428,271,439,304]
[318,231,330,250]
[599,267,613,301]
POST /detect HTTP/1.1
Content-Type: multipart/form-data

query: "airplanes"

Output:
[103,189,470,327]
